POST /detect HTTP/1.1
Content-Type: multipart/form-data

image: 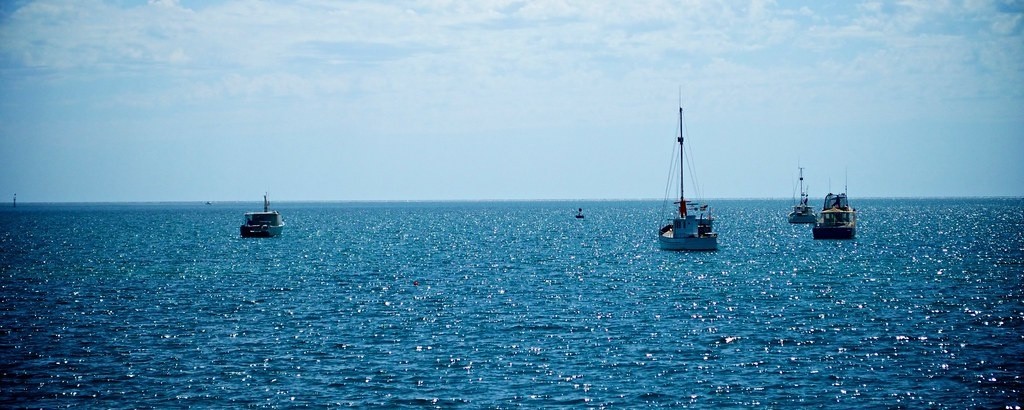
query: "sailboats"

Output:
[658,108,718,252]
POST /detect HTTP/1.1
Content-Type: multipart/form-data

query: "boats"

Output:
[812,192,856,240]
[240,194,285,238]
[788,167,816,224]
